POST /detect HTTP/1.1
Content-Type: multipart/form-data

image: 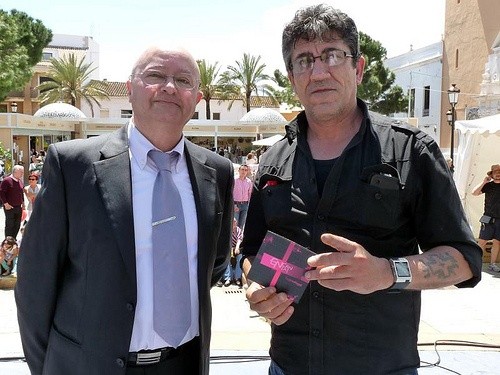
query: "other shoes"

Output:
[488,263,500,271]
[216,281,223,287]
[224,280,231,287]
[235,279,241,285]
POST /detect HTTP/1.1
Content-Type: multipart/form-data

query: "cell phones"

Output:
[370,175,400,190]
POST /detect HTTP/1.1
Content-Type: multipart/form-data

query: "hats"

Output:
[487,165,500,177]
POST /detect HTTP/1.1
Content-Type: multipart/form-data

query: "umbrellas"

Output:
[252,134,284,146]
[35,102,86,121]
[241,107,286,125]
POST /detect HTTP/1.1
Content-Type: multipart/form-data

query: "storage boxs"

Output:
[475,238,500,263]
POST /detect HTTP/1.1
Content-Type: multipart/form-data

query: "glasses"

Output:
[132,69,202,91]
[29,179,36,180]
[291,48,354,75]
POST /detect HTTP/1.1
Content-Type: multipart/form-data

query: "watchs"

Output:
[386,257,412,294]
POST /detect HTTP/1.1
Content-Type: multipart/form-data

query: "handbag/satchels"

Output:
[479,215,495,230]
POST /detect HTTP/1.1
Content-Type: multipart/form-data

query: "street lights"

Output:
[446,83,461,176]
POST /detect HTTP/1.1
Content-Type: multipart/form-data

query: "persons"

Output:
[218,149,224,157]
[246,146,267,164]
[32,150,46,163]
[0,165,24,240]
[232,164,253,231]
[239,3,484,375]
[224,218,243,286]
[0,236,18,276]
[471,164,500,272]
[24,173,41,222]
[15,43,235,375]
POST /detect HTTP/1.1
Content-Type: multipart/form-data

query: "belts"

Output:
[127,338,201,365]
[234,201,248,204]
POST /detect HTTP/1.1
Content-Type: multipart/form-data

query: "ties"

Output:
[148,150,192,349]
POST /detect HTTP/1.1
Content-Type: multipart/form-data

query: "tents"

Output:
[454,114,500,239]
